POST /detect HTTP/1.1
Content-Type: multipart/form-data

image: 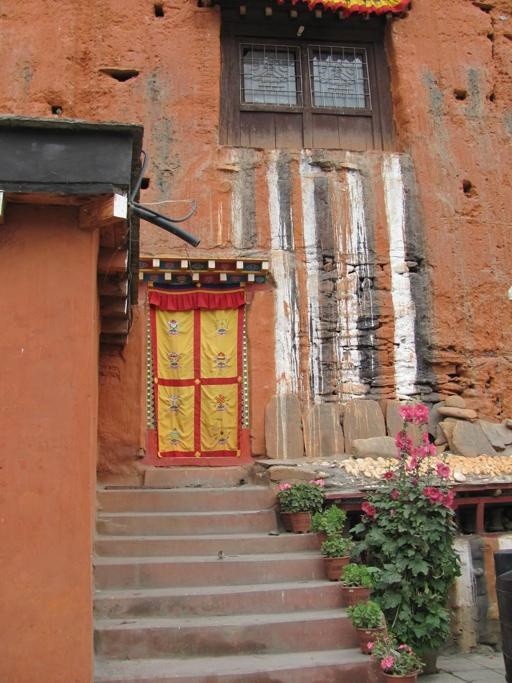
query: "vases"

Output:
[282,509,312,534]
[412,647,438,675]
[380,668,420,683]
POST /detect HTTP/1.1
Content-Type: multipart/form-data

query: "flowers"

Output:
[365,631,428,683]
[348,401,463,648]
[275,479,326,513]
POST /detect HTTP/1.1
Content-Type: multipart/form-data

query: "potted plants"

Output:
[311,503,387,653]
